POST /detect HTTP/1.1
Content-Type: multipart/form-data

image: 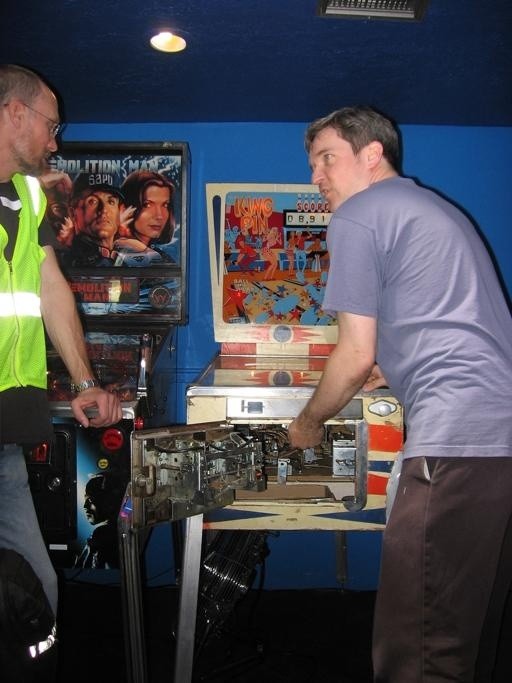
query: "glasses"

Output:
[20,101,63,135]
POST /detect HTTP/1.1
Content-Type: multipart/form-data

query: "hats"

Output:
[72,161,126,202]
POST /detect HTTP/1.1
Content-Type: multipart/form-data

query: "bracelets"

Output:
[70,377,99,393]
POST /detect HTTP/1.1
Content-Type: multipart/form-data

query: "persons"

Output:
[72,468,126,568]
[114,167,178,263]
[37,165,73,269]
[1,65,124,679]
[287,107,511,682]
[66,164,144,266]
